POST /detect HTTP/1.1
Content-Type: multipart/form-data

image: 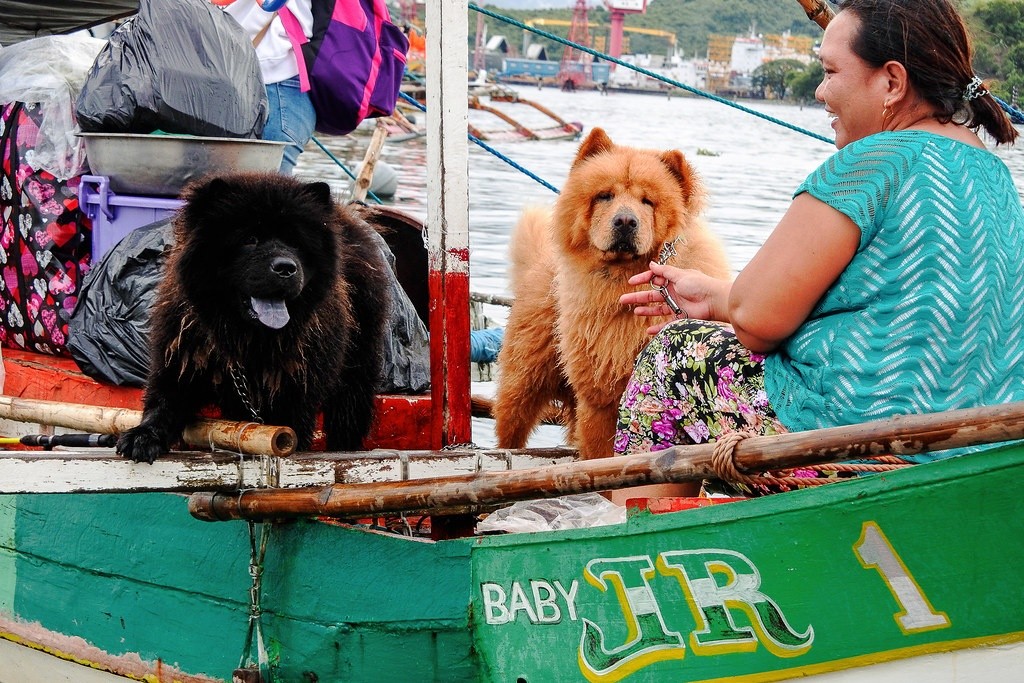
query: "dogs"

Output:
[115,165,394,465]
[490,128,732,461]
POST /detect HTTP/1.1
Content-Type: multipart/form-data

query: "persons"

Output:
[612,1,1024,504]
[206,0,319,177]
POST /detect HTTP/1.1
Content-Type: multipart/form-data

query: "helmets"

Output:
[347,159,398,196]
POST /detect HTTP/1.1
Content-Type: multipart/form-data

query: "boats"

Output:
[0,0,1024,683]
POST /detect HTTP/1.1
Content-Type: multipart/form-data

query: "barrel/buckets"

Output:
[80,175,189,268]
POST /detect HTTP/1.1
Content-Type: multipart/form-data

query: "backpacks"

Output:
[258,0,409,135]
[0,99,93,358]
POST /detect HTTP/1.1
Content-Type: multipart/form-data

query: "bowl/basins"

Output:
[75,132,297,199]
